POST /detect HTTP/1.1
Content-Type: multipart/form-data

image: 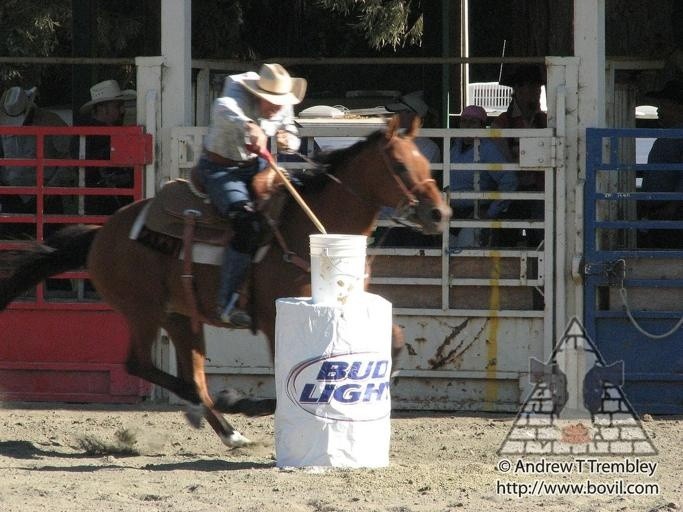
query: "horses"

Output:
[0,111,454,452]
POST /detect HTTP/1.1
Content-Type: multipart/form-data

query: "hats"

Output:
[385,95,428,119]
[0,86,37,139]
[498,64,546,85]
[461,106,487,126]
[239,63,307,107]
[80,80,137,114]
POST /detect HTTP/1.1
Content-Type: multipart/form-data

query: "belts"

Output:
[203,149,259,166]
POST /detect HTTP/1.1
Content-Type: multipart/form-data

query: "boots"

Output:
[216,244,253,330]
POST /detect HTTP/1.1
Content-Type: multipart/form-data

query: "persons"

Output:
[632,78,683,251]
[440,104,520,249]
[0,82,74,274]
[499,69,547,246]
[185,61,308,329]
[73,78,139,228]
[366,92,441,249]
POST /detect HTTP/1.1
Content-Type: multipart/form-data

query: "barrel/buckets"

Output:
[308,233,369,302]
[274,296,394,471]
